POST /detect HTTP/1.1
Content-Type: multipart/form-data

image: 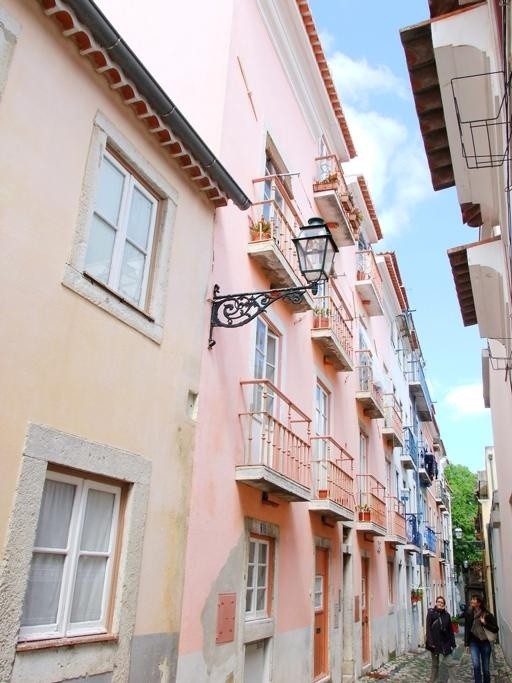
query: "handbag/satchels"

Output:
[483,626,497,642]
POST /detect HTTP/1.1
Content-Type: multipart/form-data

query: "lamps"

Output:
[209,216,340,347]
[453,524,462,539]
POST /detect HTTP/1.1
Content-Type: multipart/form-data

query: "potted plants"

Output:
[313,307,331,329]
[313,172,340,192]
[358,504,372,521]
[451,615,463,633]
[341,192,354,212]
[411,591,423,602]
[249,213,278,242]
[349,207,363,229]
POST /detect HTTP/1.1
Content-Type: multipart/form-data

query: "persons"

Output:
[464,595,499,683]
[425,595,457,683]
